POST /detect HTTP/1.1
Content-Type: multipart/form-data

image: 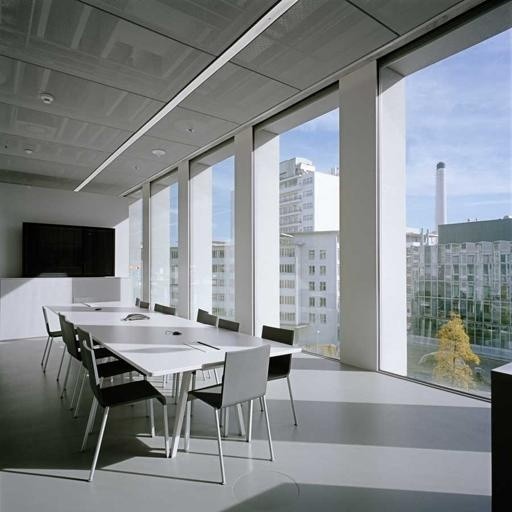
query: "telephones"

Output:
[123,314,151,321]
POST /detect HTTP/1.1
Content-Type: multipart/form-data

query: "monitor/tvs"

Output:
[22,221,116,278]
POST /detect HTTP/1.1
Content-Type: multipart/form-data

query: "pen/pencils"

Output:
[82,302,91,307]
[197,342,220,350]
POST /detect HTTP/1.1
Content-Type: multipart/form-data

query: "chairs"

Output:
[183,343,275,486]
[134,297,151,309]
[152,303,178,315]
[80,339,171,483]
[73,326,150,433]
[195,307,218,327]
[40,304,62,376]
[218,318,240,332]
[60,320,113,401]
[56,311,100,383]
[239,325,297,443]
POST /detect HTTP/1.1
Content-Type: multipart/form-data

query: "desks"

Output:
[491,362,512,512]
[48,301,304,459]
